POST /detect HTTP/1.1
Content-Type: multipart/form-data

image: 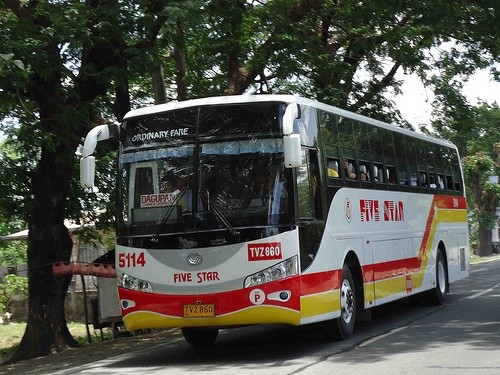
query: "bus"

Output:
[78,94,471,348]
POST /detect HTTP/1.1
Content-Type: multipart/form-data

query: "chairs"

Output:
[178,161,441,216]
